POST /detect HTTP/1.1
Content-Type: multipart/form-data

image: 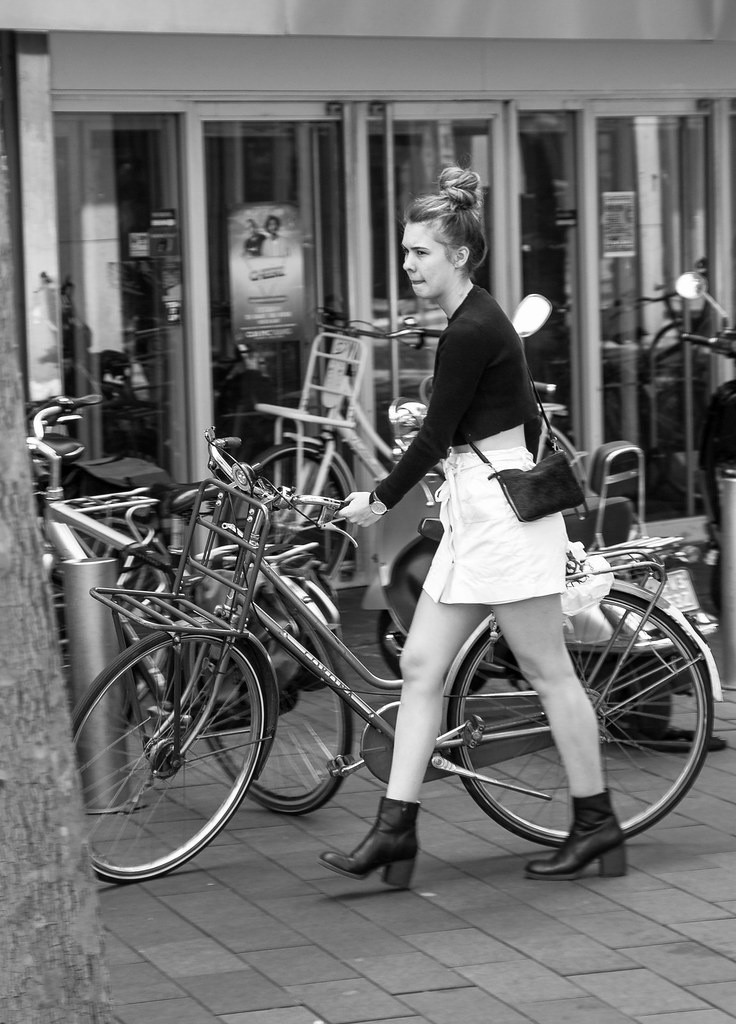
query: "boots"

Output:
[319,797,421,888]
[522,790,627,881]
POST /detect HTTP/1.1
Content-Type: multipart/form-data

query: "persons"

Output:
[318,168,629,888]
[243,215,290,257]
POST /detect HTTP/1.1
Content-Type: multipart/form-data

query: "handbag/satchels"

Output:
[488,451,586,522]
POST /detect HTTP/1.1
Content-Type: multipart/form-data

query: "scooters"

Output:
[362,291,720,744]
[675,270,736,605]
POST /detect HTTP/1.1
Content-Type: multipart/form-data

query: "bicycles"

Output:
[71,424,723,885]
[26,310,586,818]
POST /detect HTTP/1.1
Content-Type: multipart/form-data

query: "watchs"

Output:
[369,491,389,515]
